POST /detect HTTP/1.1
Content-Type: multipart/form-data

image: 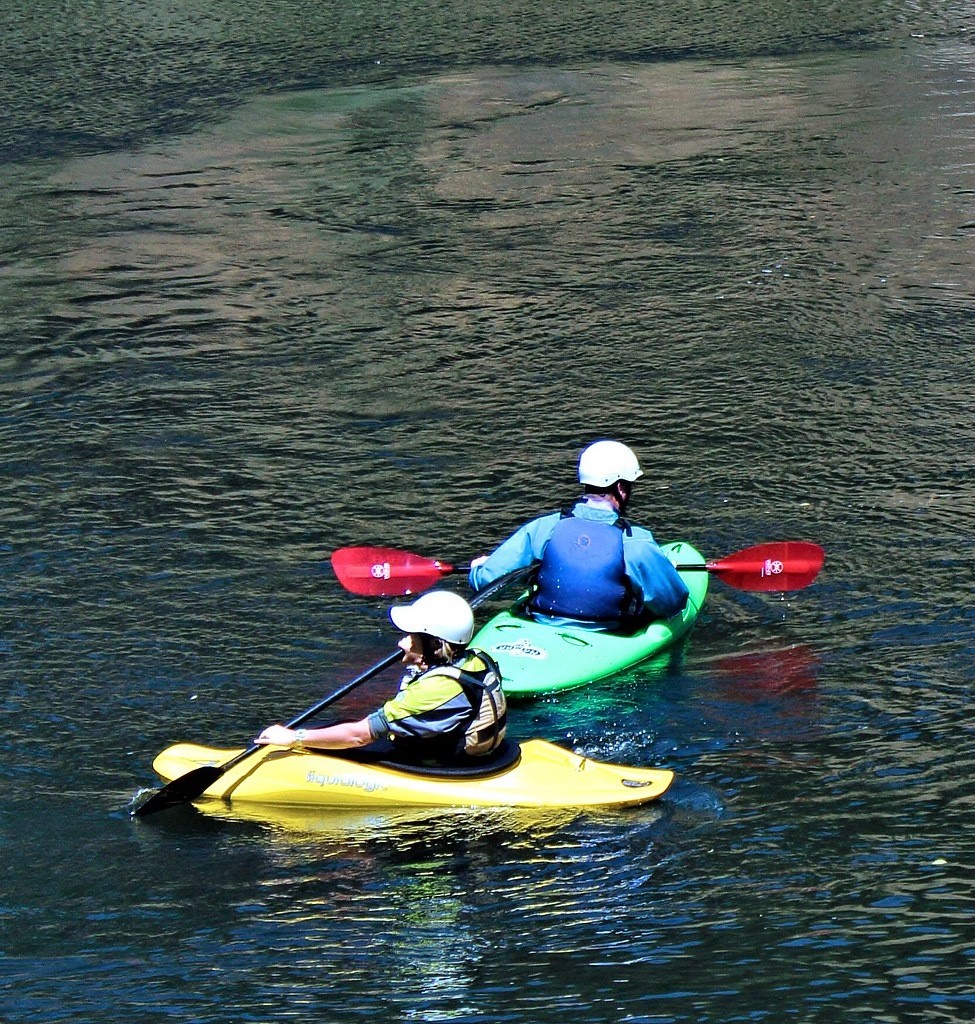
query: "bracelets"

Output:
[294,729,306,750]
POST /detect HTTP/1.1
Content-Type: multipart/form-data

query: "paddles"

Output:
[329,541,828,598]
[132,561,548,820]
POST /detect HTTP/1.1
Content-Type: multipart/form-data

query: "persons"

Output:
[254,590,508,767]
[468,440,689,637]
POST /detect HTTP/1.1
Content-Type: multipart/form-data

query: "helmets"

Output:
[390,591,474,644]
[579,441,644,487]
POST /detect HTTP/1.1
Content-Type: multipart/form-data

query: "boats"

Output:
[151,739,676,830]
[467,542,709,698]
